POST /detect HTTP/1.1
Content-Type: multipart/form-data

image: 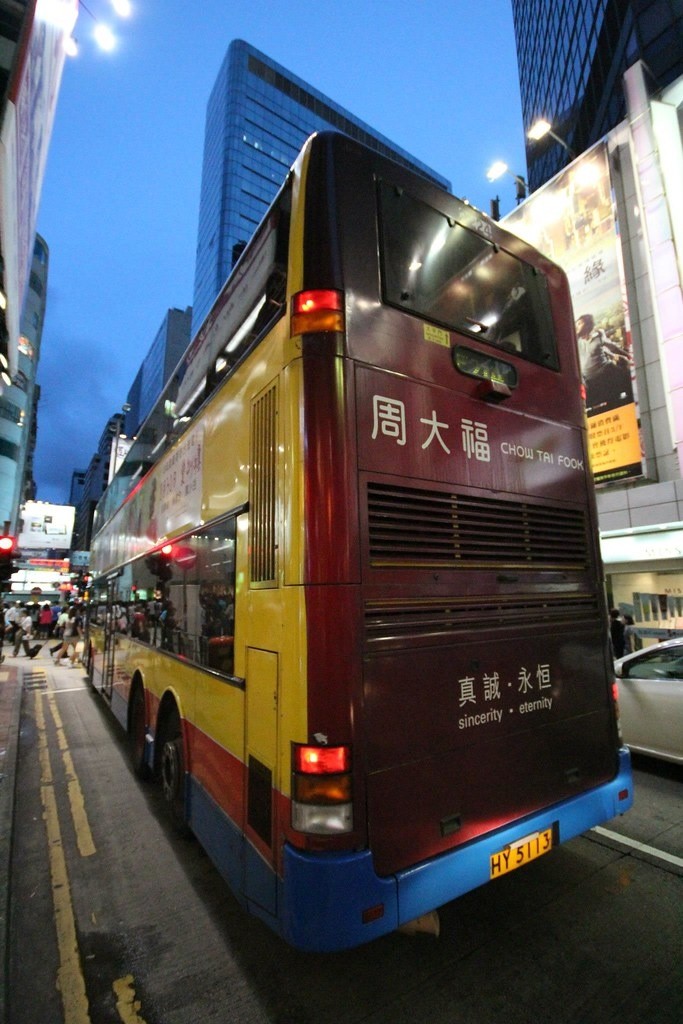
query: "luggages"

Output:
[27,636,51,659]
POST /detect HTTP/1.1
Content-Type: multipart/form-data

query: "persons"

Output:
[87,574,235,668]
[0,593,87,668]
[573,313,632,412]
[609,607,638,659]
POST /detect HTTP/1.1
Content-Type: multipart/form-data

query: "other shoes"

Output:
[54,661,64,666]
[71,664,81,668]
[61,655,68,658]
[49,648,53,656]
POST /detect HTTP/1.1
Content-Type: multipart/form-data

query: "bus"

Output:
[74,129,637,958]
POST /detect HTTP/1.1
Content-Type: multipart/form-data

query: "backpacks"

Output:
[634,632,642,651]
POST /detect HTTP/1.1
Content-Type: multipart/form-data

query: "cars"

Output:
[612,637,683,769]
